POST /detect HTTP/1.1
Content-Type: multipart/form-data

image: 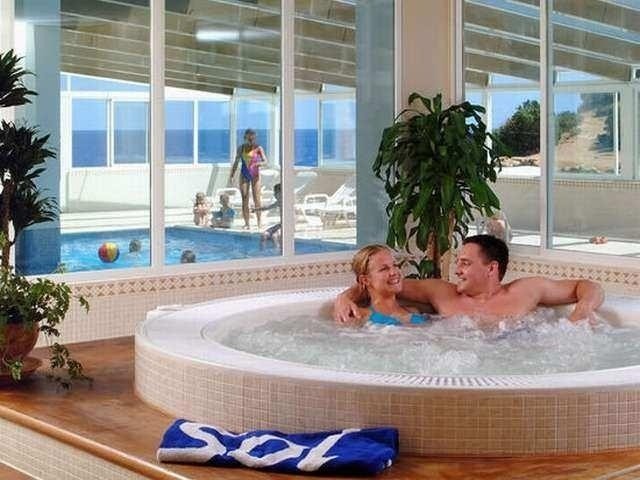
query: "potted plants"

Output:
[0,50,96,387]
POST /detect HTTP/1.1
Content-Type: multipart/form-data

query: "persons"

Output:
[193,191,213,228]
[179,250,199,263]
[128,239,142,254]
[209,194,235,231]
[344,242,431,331]
[229,126,268,230]
[331,233,606,351]
[248,181,283,254]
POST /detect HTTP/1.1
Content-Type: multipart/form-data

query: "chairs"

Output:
[216,167,356,232]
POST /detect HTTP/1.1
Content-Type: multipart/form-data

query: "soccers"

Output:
[99,242,119,262]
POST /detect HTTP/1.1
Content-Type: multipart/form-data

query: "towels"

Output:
[155,419,399,474]
